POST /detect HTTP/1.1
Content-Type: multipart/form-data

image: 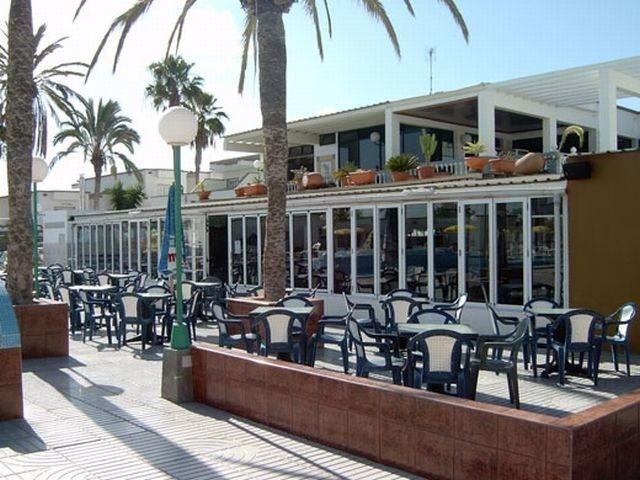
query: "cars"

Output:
[36,244,44,266]
[0,248,7,272]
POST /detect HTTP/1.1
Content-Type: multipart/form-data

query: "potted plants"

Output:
[333,160,359,185]
[417,133,437,179]
[490,150,517,174]
[291,166,310,190]
[235,174,266,196]
[384,153,419,181]
[347,168,376,186]
[463,140,496,171]
[195,182,210,200]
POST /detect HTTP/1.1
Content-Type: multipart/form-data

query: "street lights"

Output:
[159,104,201,403]
[31,155,49,301]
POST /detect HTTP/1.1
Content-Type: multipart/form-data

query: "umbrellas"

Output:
[382,209,398,262]
[158,182,188,305]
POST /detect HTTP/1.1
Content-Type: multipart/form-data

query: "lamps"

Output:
[370,132,384,146]
[254,160,264,172]
